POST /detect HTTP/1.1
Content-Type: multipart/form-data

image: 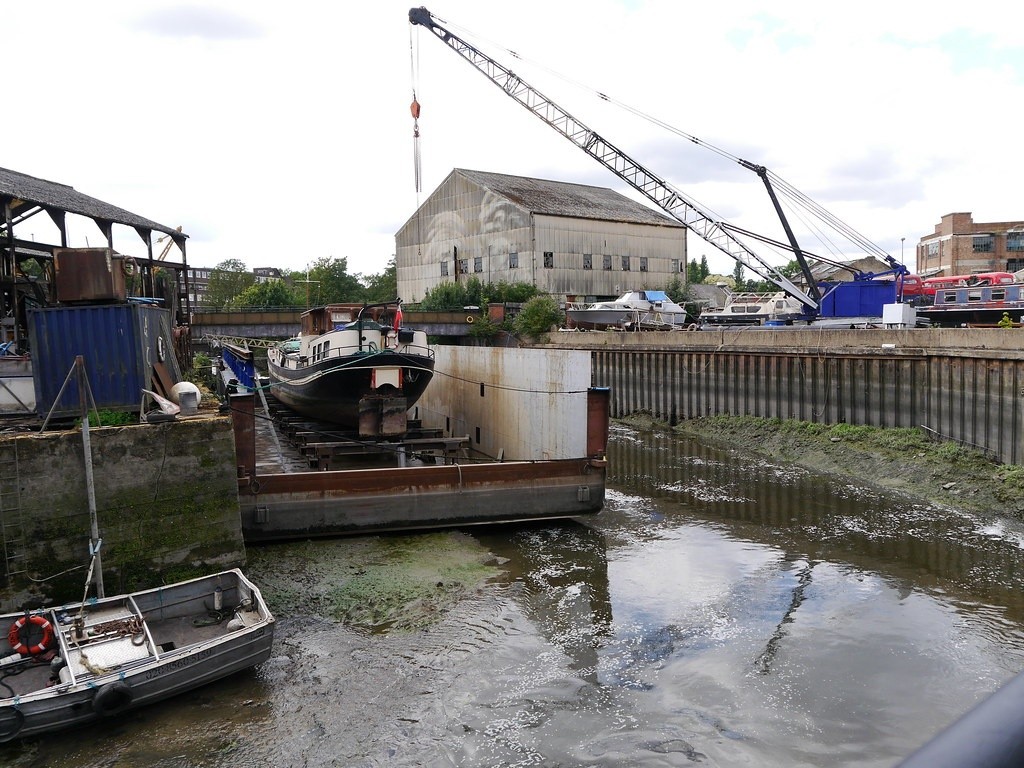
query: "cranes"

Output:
[408,6,910,329]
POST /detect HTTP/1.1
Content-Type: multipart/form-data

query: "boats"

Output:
[267,298,435,411]
[915,285,1024,322]
[699,292,803,326]
[566,290,687,330]
[0,567,276,742]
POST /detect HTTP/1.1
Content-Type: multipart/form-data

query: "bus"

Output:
[872,275,922,302]
[923,273,1013,297]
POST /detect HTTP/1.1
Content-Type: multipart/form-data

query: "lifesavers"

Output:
[466,316,474,323]
[8,616,54,655]
[93,682,131,718]
[384,334,400,350]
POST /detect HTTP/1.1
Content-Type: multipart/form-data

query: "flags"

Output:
[394,305,403,332]
